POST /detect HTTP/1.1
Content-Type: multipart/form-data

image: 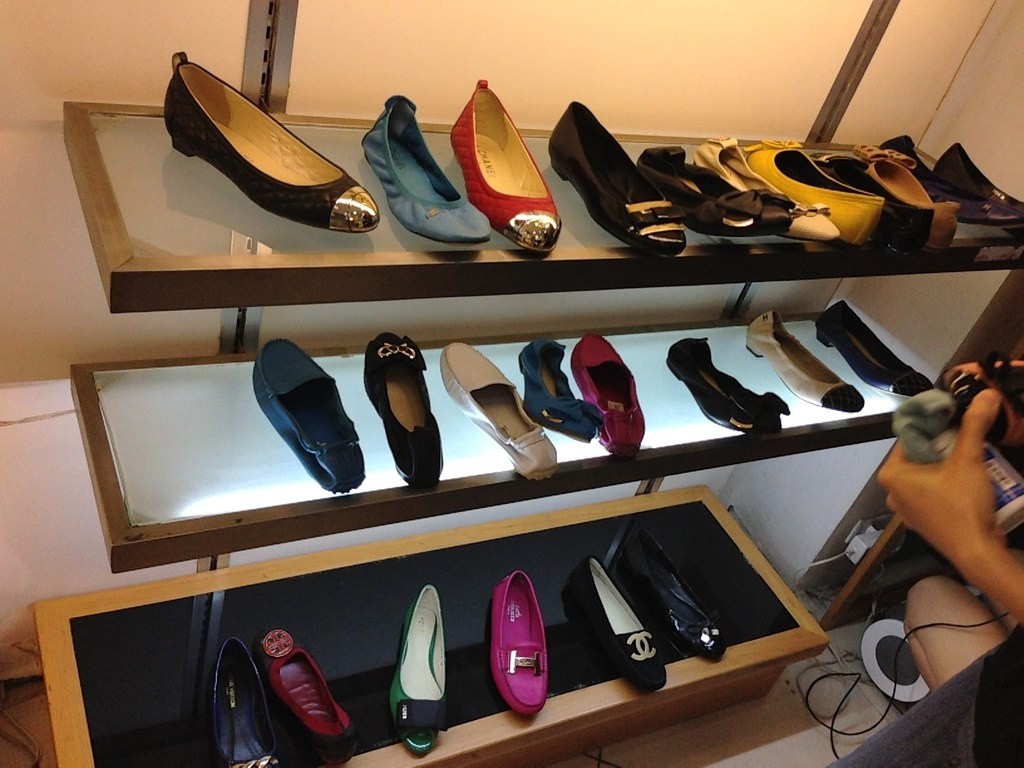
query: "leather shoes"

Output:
[879,134,1024,229]
[206,637,281,768]
[637,146,796,237]
[621,528,727,659]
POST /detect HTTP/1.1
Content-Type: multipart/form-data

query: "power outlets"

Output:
[845,513,893,544]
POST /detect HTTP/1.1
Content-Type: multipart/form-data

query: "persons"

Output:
[876,389,1024,692]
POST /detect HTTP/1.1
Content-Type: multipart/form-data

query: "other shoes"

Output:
[451,80,562,252]
[364,332,443,489]
[571,332,646,460]
[164,52,380,233]
[362,95,492,245]
[389,581,449,755]
[745,310,864,412]
[743,139,886,248]
[519,338,603,443]
[692,136,840,243]
[569,554,666,691]
[853,143,960,255]
[252,338,366,494]
[667,337,790,434]
[815,300,933,398]
[490,570,548,717]
[811,152,934,258]
[255,624,359,765]
[440,341,557,481]
[549,101,686,255]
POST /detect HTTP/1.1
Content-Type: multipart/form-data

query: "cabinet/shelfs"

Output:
[30,102,1024,768]
